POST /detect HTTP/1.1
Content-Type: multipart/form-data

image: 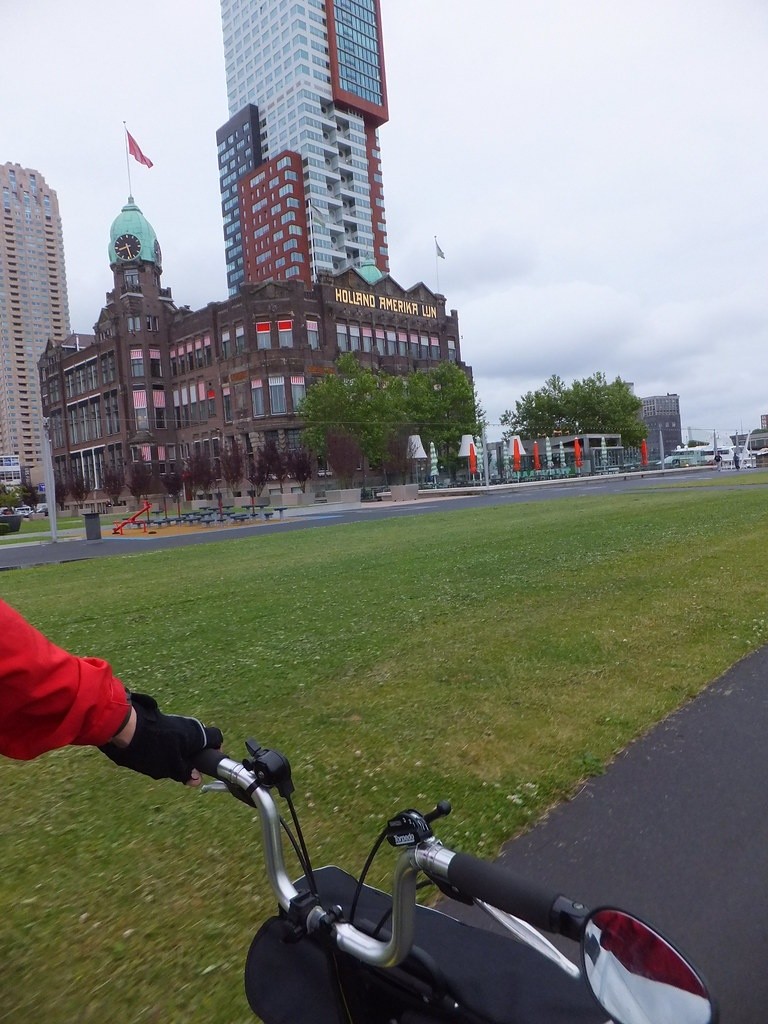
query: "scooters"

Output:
[192,733,723,1024]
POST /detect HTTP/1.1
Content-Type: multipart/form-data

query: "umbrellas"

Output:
[513,439,521,482]
[546,437,554,480]
[477,437,484,485]
[600,435,610,474]
[430,442,440,488]
[533,441,542,470]
[469,443,477,486]
[503,441,509,483]
[559,441,567,468]
[574,437,584,477]
[641,438,650,470]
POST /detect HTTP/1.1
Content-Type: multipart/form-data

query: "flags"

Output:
[128,133,154,169]
[436,241,445,259]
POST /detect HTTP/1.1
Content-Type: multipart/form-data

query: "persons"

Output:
[714,453,723,471]
[733,453,740,472]
[0,596,225,788]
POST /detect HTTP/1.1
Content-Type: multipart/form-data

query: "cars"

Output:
[35,506,49,516]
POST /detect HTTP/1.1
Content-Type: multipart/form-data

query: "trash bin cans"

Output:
[81,512,103,540]
